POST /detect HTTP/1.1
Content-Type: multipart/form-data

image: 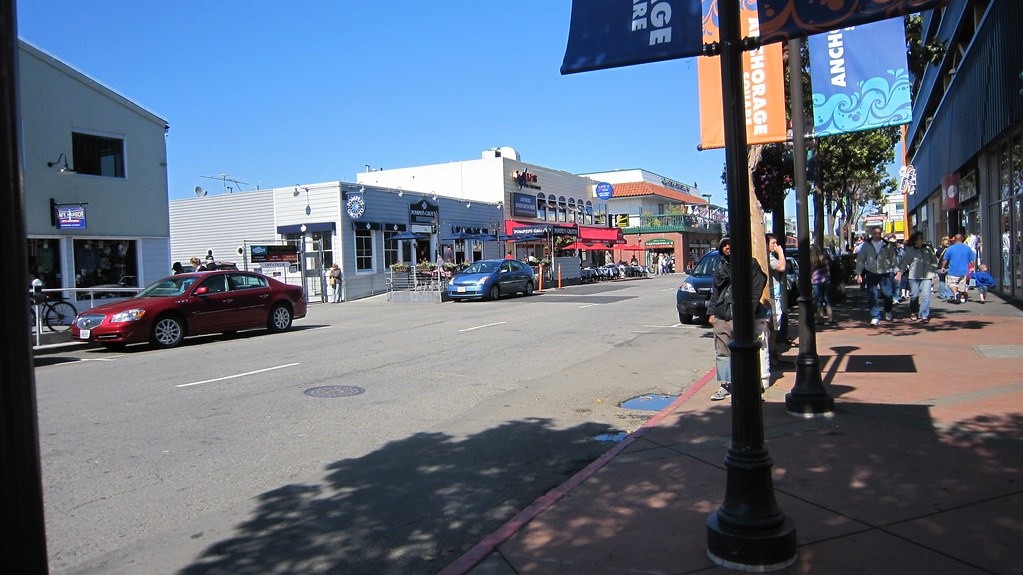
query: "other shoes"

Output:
[922,317,929,322]
[954,292,965,304]
[911,313,917,321]
[760,375,770,390]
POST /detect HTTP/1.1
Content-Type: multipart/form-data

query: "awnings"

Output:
[505,221,627,244]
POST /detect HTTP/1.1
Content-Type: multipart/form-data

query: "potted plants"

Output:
[389,260,471,273]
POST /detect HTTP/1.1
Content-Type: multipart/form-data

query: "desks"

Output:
[580,266,647,283]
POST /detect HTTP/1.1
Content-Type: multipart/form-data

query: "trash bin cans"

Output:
[756,318,770,390]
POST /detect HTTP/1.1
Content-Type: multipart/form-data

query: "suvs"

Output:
[677,248,721,324]
[172,262,242,291]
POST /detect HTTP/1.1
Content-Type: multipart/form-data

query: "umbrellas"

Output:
[562,241,646,281]
[385,230,431,290]
[441,231,541,271]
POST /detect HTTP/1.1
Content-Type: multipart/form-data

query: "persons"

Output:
[505,250,514,259]
[706,233,768,401]
[528,252,540,263]
[172,258,225,292]
[652,255,672,275]
[765,233,797,347]
[809,244,834,325]
[329,263,341,303]
[845,227,995,326]
[1001,228,1010,287]
[616,260,623,267]
[631,255,638,266]
[970,264,995,304]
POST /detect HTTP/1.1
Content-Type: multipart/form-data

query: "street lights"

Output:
[702,194,711,251]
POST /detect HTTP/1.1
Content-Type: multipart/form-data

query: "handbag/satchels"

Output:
[330,276,334,284]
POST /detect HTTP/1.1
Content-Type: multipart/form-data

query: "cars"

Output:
[447,258,535,301]
[71,270,307,350]
[85,275,138,299]
[786,247,830,304]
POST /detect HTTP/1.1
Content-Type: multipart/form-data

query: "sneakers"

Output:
[710,382,732,400]
[870,319,881,325]
[885,312,892,320]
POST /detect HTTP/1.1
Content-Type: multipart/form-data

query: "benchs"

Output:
[408,272,447,290]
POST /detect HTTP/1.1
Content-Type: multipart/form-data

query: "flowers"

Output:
[552,234,573,246]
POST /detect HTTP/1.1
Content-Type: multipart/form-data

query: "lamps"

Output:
[391,186,405,197]
[458,199,472,208]
[948,67,957,75]
[488,202,502,210]
[424,191,438,201]
[48,153,77,175]
[294,184,309,196]
[927,116,934,121]
[351,182,366,194]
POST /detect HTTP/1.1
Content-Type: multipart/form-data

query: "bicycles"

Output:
[29,292,78,332]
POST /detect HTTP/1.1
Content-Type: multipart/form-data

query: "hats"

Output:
[718,237,730,255]
